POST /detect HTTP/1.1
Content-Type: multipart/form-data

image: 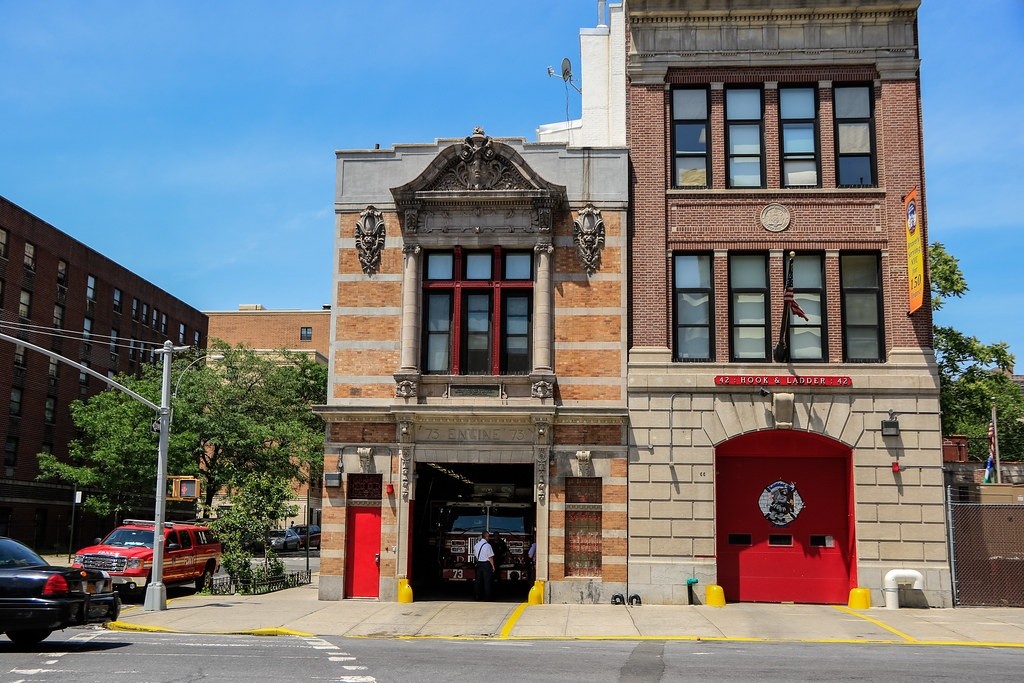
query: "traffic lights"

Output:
[179,479,200,498]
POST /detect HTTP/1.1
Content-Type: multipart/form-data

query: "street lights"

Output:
[175,355,225,400]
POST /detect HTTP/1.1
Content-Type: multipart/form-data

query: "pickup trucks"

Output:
[69,518,221,601]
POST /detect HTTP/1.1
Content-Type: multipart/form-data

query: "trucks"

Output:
[435,500,532,585]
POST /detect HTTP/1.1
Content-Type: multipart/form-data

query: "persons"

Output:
[488,531,507,582]
[290,521,294,527]
[474,531,496,602]
[528,543,536,570]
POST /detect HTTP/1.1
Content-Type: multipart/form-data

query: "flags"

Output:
[984,420,996,483]
[783,258,809,321]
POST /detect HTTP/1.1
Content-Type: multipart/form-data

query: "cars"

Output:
[266,529,302,552]
[291,524,321,550]
[0,537,123,646]
